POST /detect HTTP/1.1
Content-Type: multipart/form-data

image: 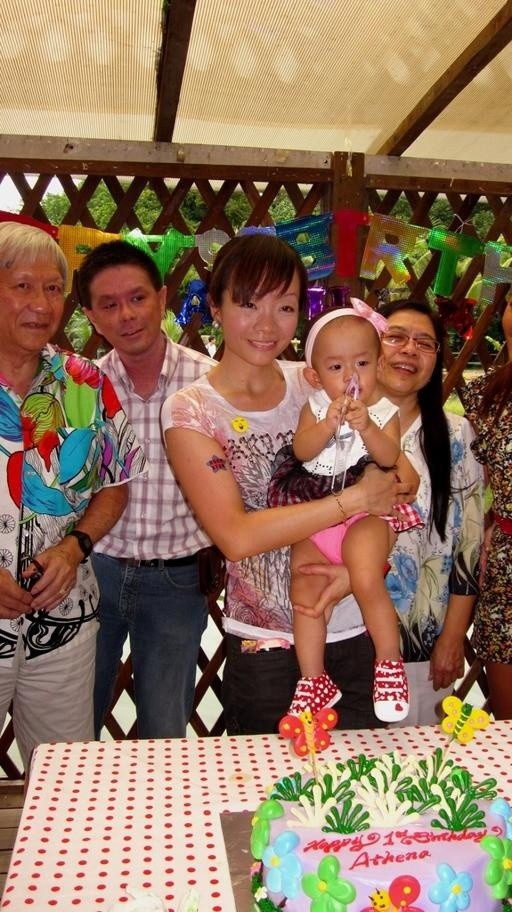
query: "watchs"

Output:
[65,529,92,565]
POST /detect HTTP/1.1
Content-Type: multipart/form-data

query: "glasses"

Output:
[380,329,440,354]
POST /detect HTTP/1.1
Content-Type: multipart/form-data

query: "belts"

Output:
[93,546,199,570]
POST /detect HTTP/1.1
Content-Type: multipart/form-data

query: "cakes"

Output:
[250,698,512,912]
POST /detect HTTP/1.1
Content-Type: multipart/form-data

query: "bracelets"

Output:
[332,494,347,526]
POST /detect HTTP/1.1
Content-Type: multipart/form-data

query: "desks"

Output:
[1,716,512,912]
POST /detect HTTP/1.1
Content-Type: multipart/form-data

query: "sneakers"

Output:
[286,669,342,720]
[372,654,411,723]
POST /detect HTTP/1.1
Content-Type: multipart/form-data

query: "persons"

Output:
[2,216,149,771]
[73,238,221,737]
[262,305,409,734]
[461,289,511,722]
[157,232,420,735]
[367,289,491,731]
